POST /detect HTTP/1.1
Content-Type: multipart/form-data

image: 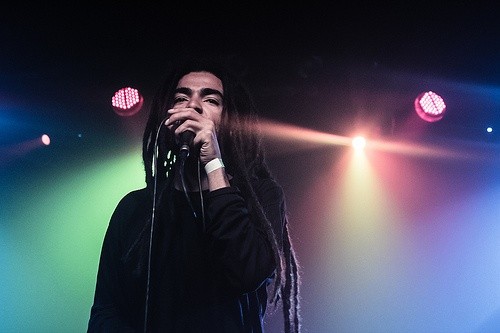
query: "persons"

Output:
[85,54,301,333]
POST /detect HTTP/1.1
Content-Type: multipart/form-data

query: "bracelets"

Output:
[204,157,225,175]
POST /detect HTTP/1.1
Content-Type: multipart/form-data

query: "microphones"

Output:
[178,125,195,174]
